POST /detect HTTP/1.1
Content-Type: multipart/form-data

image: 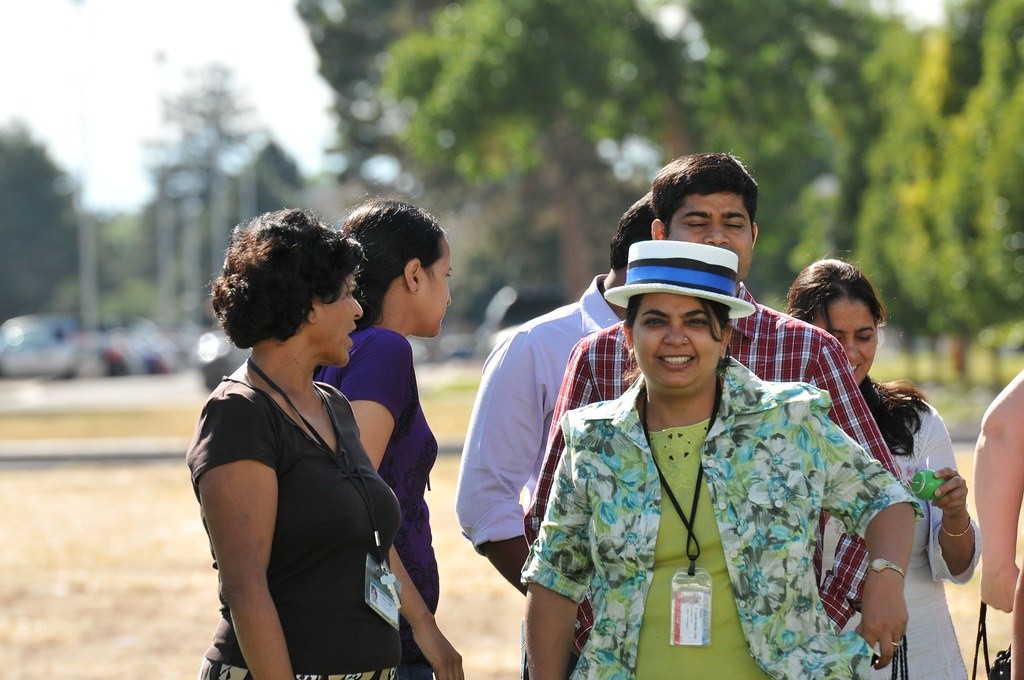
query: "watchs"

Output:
[865,558,906,581]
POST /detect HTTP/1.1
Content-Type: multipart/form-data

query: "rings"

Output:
[892,637,904,647]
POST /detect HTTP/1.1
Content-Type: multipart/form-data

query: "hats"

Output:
[603,241,757,319]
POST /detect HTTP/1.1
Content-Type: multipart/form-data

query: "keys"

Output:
[380,568,402,611]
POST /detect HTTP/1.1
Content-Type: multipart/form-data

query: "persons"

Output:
[456,191,664,593]
[523,240,923,680]
[784,258,980,679]
[313,197,466,679]
[524,150,901,680]
[972,368,1024,680]
[186,207,403,679]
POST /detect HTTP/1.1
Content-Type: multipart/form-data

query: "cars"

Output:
[0,311,259,392]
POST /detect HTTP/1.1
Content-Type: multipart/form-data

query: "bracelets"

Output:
[942,517,972,538]
